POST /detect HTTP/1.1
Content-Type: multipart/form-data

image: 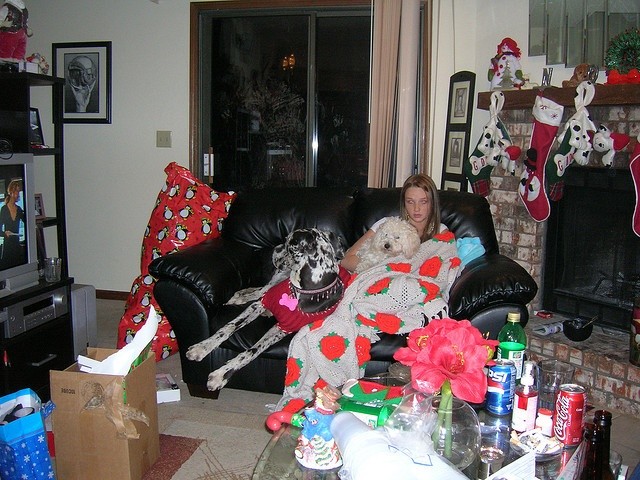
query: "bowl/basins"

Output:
[564,320,592,341]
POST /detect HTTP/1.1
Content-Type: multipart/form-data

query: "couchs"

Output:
[147,185,537,401]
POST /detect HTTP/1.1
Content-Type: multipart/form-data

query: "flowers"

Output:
[394,318,498,460]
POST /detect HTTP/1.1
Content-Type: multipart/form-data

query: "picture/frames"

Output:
[29,107,45,146]
[33,193,45,220]
[51,41,112,124]
[441,71,476,194]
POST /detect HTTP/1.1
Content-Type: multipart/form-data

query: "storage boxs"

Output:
[48,347,160,479]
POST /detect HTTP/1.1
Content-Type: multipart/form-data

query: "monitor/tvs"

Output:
[0,153,39,298]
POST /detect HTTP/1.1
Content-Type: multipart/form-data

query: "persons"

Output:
[340,174,450,275]
[0,177,26,267]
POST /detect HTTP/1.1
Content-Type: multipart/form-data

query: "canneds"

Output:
[485,358,516,416]
[630,307,640,368]
[553,384,586,449]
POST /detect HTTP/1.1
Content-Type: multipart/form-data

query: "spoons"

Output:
[583,314,601,328]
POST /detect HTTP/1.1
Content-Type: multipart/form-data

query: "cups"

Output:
[536,360,575,410]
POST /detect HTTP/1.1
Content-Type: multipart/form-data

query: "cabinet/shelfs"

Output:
[1,71,69,283]
[0,277,74,403]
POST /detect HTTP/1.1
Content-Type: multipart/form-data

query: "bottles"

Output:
[580,425,604,480]
[44,257,62,282]
[499,313,526,386]
[593,412,618,480]
[535,409,554,436]
[512,361,539,432]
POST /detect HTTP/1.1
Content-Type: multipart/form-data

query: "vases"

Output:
[406,396,481,471]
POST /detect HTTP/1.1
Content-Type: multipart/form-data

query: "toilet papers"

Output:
[331,412,389,473]
